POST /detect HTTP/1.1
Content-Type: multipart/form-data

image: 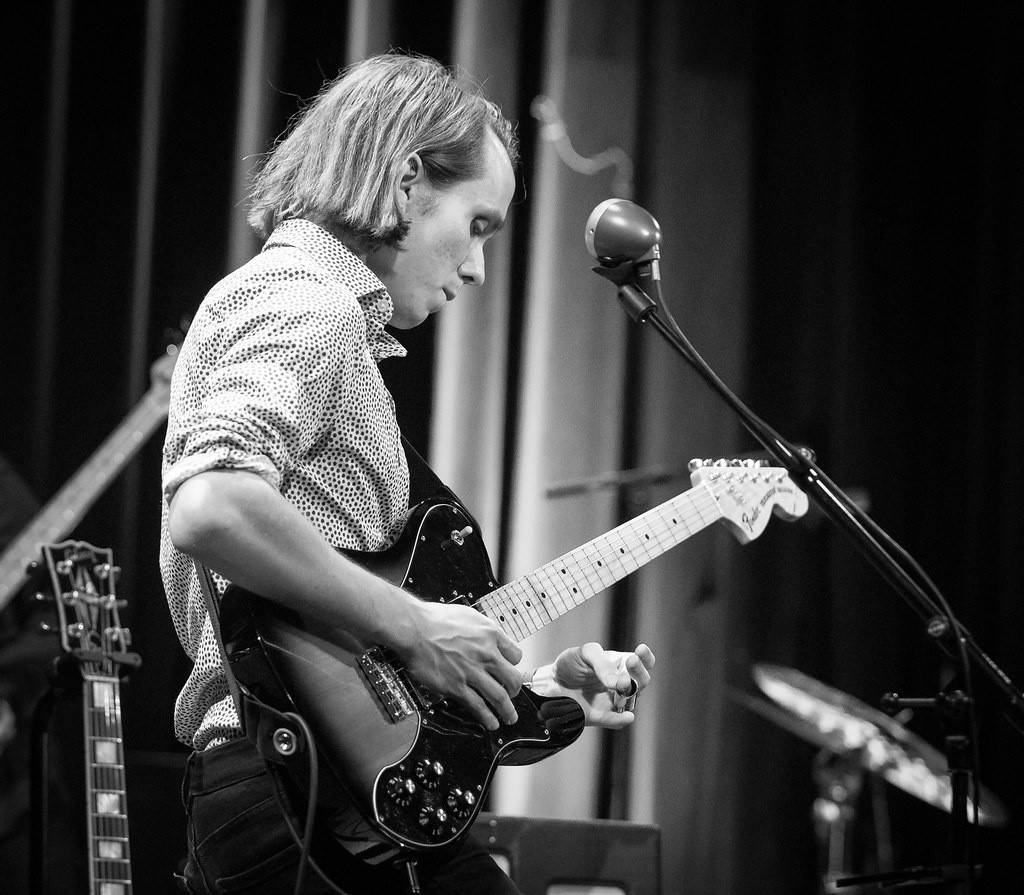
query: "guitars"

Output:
[210,450,810,894]
[23,536,148,894]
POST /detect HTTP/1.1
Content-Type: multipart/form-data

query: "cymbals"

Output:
[730,655,1008,831]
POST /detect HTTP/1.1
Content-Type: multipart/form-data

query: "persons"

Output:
[0,457,77,895]
[159,53,655,895]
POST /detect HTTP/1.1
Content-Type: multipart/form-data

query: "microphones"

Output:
[586,198,662,269]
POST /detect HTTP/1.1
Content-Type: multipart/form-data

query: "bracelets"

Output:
[523,669,535,690]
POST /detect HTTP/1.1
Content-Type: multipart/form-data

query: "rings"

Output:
[615,678,638,699]
